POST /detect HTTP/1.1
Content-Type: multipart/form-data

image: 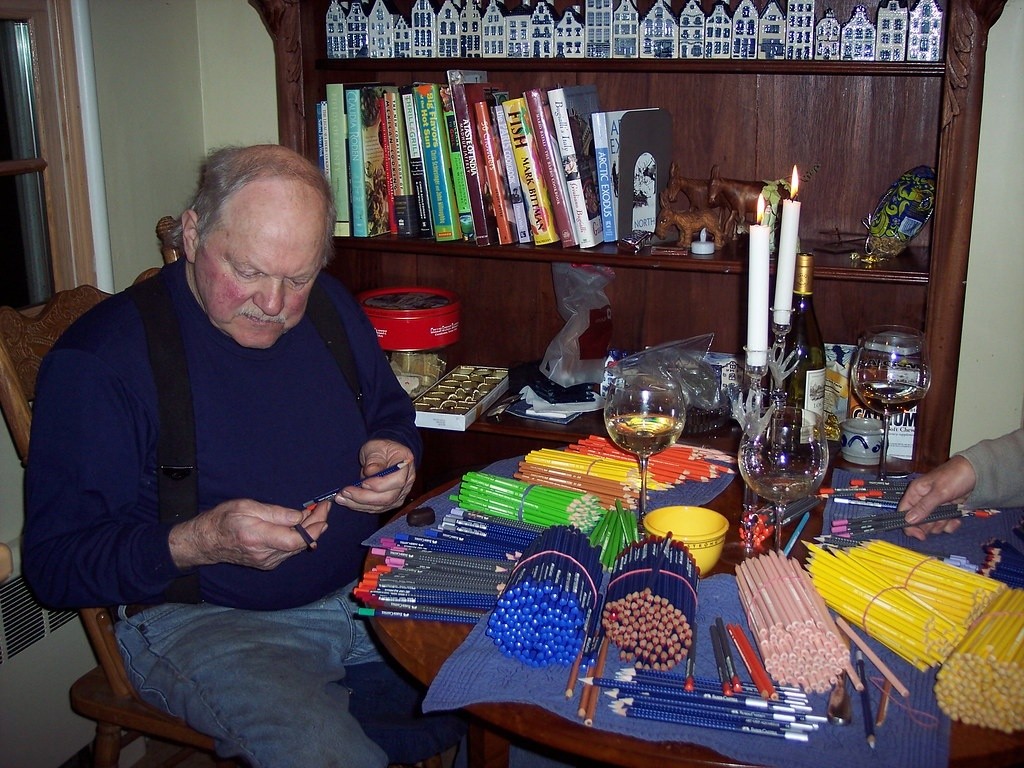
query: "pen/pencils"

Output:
[781,512,810,560]
[739,493,822,547]
[709,616,779,701]
[448,469,606,536]
[514,434,737,513]
[834,614,910,696]
[484,522,608,727]
[590,499,638,573]
[804,536,1024,741]
[822,477,1000,536]
[854,648,877,749]
[294,523,318,550]
[350,508,549,625]
[576,668,828,743]
[601,530,701,693]
[733,550,864,690]
[301,457,417,511]
[875,680,892,727]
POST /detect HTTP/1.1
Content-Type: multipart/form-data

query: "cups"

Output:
[840,419,884,465]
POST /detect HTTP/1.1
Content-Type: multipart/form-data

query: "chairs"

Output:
[0,217,461,768]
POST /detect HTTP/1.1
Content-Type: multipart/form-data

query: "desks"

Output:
[362,474,1024,768]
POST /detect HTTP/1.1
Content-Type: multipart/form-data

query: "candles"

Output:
[772,164,802,326]
[747,193,771,366]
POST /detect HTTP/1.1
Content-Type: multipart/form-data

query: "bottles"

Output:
[784,253,827,455]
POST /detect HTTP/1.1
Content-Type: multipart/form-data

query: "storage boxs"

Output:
[412,364,510,431]
[644,346,744,390]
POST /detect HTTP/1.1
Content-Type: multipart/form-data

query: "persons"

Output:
[896,426,1024,540]
[18,144,469,768]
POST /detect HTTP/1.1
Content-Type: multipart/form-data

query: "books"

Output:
[317,68,674,250]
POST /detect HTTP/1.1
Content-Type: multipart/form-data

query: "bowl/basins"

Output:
[643,505,729,578]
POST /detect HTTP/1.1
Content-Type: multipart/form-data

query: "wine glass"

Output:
[851,325,932,482]
[738,407,829,559]
[604,376,686,536]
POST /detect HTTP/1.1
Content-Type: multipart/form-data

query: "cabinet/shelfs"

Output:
[246,0,1007,500]
